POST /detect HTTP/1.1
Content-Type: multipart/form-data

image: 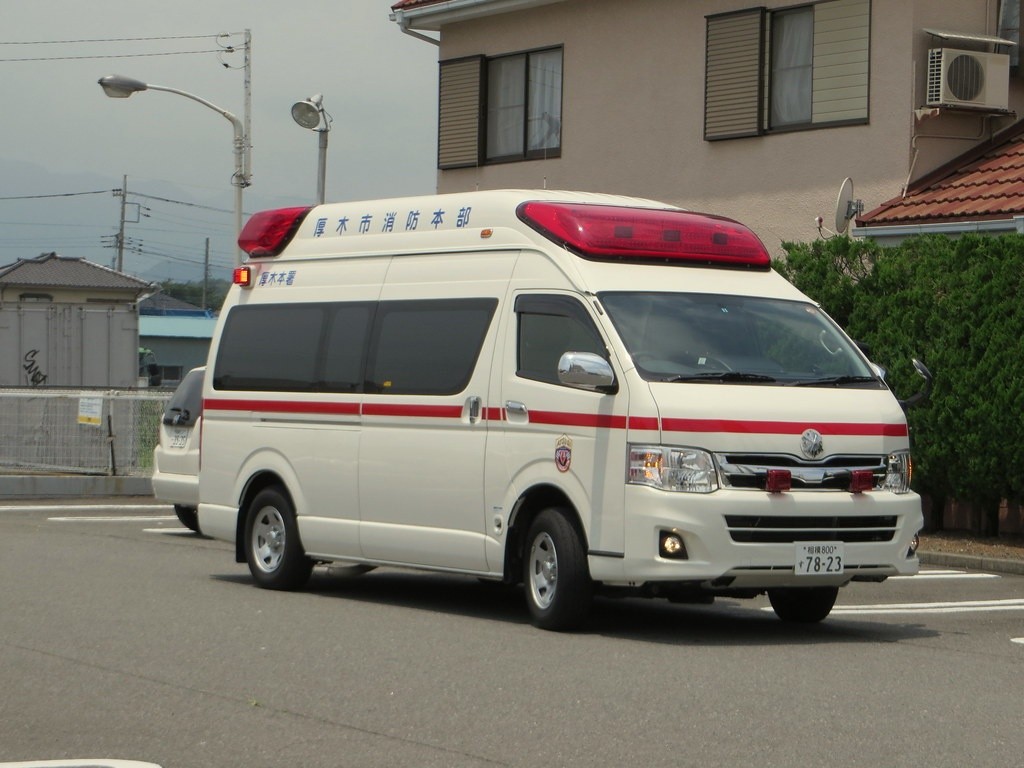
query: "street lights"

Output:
[96,72,255,266]
[291,91,334,207]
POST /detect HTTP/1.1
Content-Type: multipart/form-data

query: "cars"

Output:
[151,366,207,531]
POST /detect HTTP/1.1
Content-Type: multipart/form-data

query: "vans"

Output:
[197,187,933,632]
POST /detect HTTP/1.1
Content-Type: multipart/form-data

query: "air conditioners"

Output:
[925,47,1010,109]
